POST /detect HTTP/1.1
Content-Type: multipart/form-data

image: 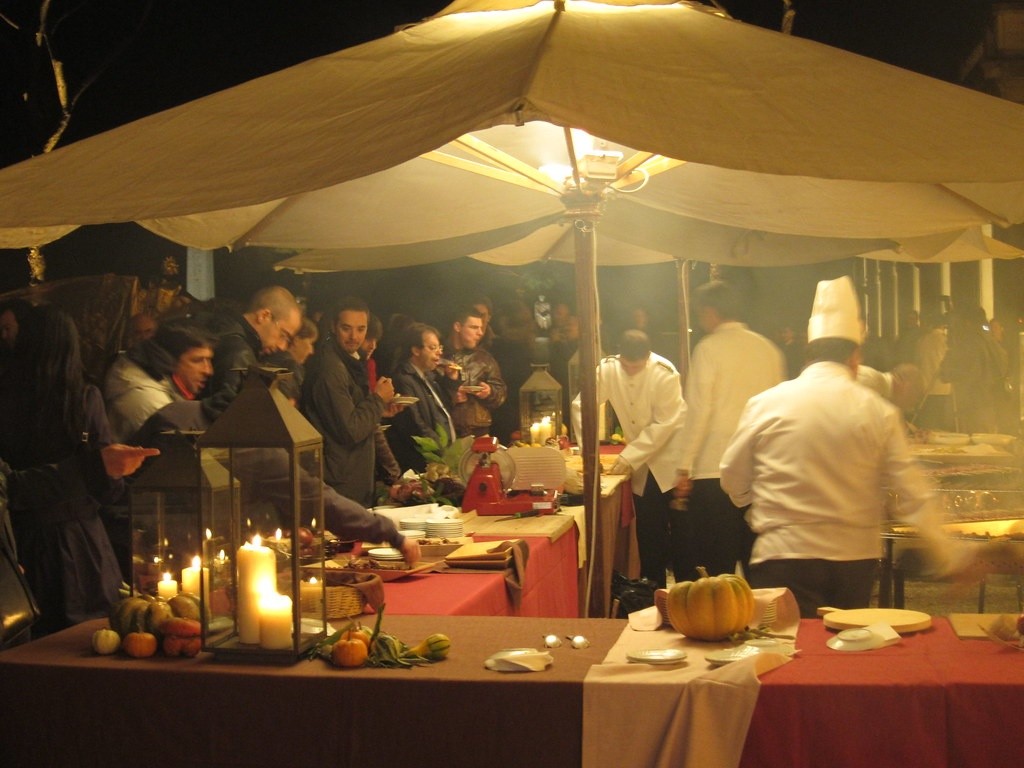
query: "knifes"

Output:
[494,510,540,522]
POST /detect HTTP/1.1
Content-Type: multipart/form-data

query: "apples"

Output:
[269,526,315,555]
[331,621,373,666]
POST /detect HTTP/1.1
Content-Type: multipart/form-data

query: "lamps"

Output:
[576,150,623,182]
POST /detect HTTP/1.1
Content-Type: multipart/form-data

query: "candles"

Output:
[236,535,322,649]
[158,536,231,605]
[530,418,552,444]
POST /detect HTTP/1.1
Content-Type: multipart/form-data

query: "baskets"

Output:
[287,568,367,620]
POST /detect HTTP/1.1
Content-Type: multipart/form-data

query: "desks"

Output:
[305,442,639,616]
[0,611,1024,768]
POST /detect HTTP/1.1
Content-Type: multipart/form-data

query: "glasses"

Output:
[270,315,291,344]
[422,345,443,352]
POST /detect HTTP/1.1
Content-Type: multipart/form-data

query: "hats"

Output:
[807,274,864,345]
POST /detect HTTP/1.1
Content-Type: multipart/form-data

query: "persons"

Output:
[717,275,966,617]
[570,331,699,589]
[3,252,1022,645]
[673,280,783,582]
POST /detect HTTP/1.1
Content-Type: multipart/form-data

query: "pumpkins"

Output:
[666,566,757,641]
[91,591,203,658]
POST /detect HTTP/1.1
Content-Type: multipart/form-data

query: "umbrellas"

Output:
[1,0,1024,616]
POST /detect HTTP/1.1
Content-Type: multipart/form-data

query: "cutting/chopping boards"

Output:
[949,614,1024,639]
[459,510,574,543]
[816,606,932,633]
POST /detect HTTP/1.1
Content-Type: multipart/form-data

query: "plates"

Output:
[393,397,419,404]
[826,628,884,651]
[933,431,1016,445]
[625,650,686,664]
[368,548,405,562]
[656,596,777,627]
[445,540,520,569]
[397,519,426,540]
[300,618,337,637]
[419,537,473,555]
[380,425,392,430]
[428,519,464,538]
[706,646,760,667]
[463,386,484,391]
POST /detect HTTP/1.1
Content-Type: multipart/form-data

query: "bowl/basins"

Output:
[330,538,363,555]
[570,447,579,455]
